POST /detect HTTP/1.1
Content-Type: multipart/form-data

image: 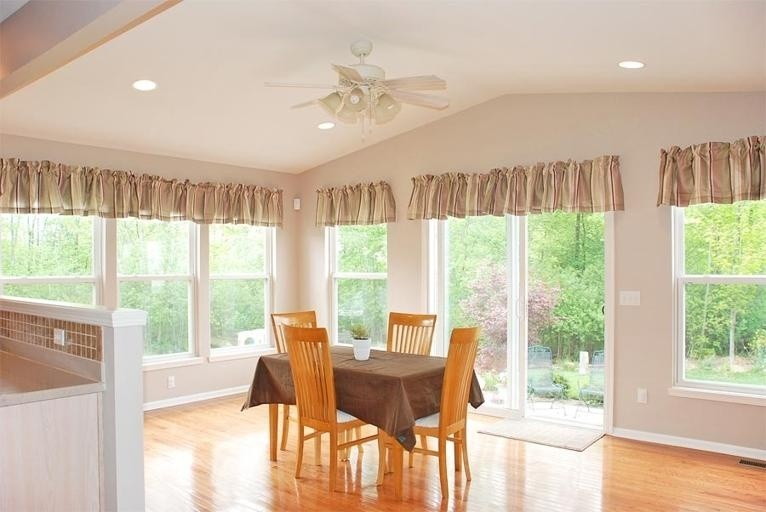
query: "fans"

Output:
[267,40,449,128]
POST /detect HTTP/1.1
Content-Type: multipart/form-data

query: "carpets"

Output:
[478,419,606,452]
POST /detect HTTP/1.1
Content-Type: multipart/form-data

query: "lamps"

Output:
[317,87,400,124]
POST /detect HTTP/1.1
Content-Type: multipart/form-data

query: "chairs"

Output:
[269,312,363,462]
[281,322,391,494]
[376,312,439,468]
[376,325,478,500]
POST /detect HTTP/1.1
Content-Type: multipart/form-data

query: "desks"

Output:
[260,344,474,497]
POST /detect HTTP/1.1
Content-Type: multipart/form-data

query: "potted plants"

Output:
[350,324,371,360]
